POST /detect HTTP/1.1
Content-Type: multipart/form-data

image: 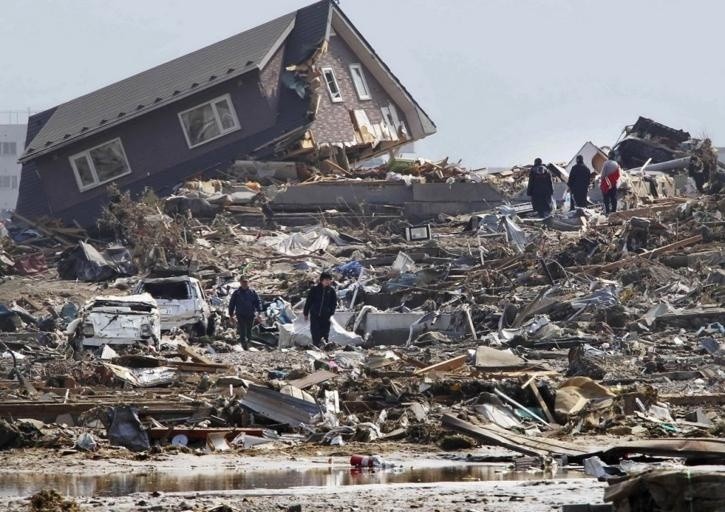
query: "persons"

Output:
[304,271,338,346]
[566,155,590,211]
[526,158,554,219]
[227,275,262,351]
[600,149,620,215]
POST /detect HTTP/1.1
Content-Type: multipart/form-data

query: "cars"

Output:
[76,275,215,356]
